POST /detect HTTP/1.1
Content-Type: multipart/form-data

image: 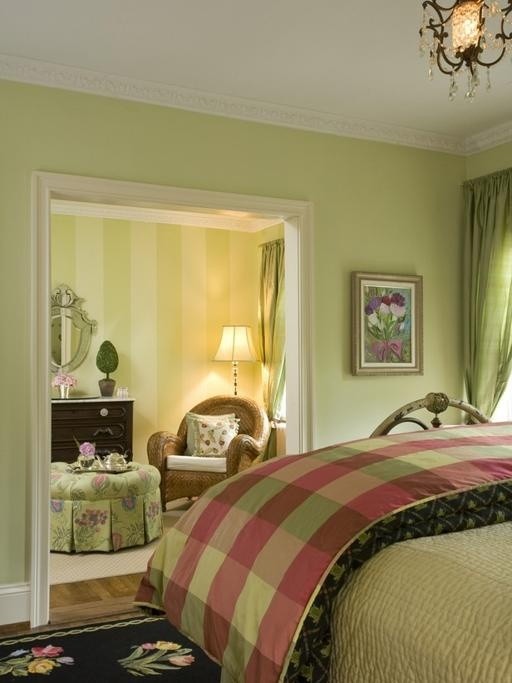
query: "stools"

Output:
[50,461,162,554]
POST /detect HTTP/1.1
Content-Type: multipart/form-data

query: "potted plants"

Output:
[95,340,118,396]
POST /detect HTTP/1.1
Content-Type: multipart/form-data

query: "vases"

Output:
[77,457,93,470]
[59,386,69,399]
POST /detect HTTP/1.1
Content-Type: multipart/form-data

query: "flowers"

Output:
[79,442,93,456]
[51,372,76,388]
[365,293,407,362]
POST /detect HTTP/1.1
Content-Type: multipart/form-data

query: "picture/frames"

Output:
[351,270,423,376]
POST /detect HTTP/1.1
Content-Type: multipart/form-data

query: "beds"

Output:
[140,393,511,681]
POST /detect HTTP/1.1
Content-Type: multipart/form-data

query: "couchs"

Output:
[147,396,272,513]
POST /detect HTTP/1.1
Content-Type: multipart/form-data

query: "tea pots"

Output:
[95,452,129,471]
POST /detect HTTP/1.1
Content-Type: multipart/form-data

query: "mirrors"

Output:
[49,282,97,373]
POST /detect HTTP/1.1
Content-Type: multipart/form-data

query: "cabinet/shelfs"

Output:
[52,398,134,462]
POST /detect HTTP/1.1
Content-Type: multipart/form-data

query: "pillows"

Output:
[190,417,238,456]
[184,412,234,456]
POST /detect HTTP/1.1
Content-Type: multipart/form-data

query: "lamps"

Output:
[213,325,257,395]
[418,0,511,99]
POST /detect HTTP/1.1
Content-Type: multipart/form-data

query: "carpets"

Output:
[50,497,201,587]
[0,611,222,682]
